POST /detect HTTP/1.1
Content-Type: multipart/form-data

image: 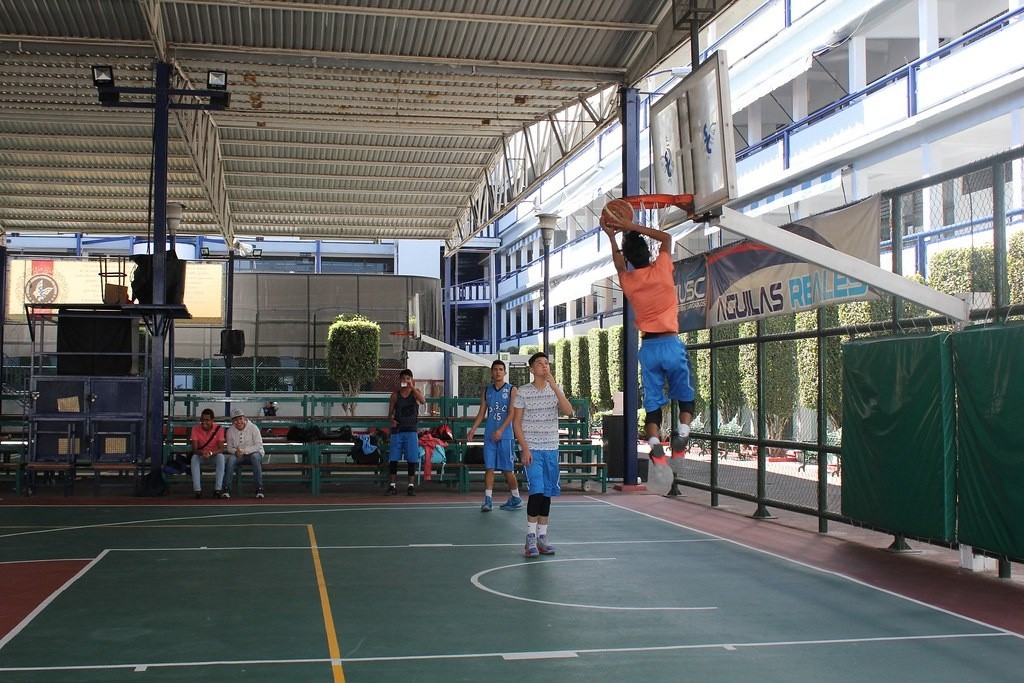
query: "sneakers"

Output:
[407,485,414,496]
[382,487,396,497]
[500,497,524,510]
[536,534,554,554]
[523,533,539,557]
[481,497,493,511]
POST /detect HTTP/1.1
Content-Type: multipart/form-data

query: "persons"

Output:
[467,360,524,512]
[189,408,225,499]
[599,211,695,458]
[383,369,426,496]
[512,353,572,556]
[221,408,265,499]
[260,401,280,434]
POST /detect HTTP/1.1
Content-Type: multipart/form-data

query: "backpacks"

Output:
[347,434,380,466]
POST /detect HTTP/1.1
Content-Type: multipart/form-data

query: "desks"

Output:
[92,464,142,493]
[29,462,73,496]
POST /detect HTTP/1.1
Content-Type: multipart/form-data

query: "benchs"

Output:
[667,420,746,459]
[162,394,608,496]
[0,419,28,497]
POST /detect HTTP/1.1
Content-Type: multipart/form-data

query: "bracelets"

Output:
[209,451,213,456]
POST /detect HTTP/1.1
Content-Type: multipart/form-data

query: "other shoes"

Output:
[214,490,221,498]
[255,492,264,499]
[649,444,665,464]
[190,490,201,500]
[221,493,231,499]
[670,433,687,453]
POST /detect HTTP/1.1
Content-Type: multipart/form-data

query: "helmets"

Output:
[228,408,244,421]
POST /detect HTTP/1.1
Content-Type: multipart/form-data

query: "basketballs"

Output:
[602,199,633,230]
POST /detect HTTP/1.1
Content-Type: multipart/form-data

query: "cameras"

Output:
[401,382,407,387]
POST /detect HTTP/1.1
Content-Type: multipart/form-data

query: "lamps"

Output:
[91,65,114,86]
[253,249,262,256]
[200,248,209,256]
[207,69,227,91]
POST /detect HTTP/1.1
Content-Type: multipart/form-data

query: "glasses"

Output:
[199,418,213,423]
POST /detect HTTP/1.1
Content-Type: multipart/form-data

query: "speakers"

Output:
[220,329,245,356]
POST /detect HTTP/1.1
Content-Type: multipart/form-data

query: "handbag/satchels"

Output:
[135,470,169,498]
[464,447,484,464]
[414,425,453,463]
[185,452,194,466]
